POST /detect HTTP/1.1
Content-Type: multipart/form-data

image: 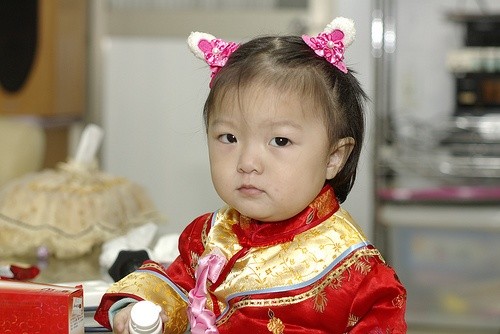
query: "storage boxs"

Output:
[0,275,85,334]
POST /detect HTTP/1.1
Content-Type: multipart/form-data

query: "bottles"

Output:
[128,301,163,334]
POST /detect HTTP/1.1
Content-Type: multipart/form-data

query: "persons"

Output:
[94,35,410,334]
